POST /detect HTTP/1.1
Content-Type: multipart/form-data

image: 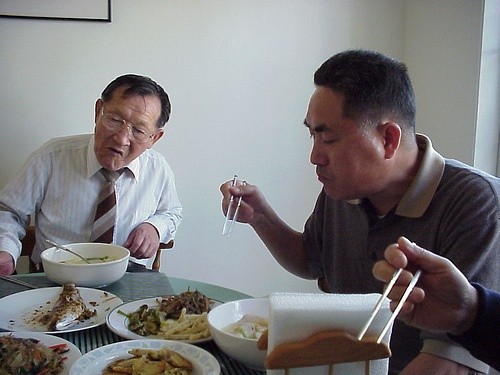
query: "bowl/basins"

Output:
[41,242,130,288]
[208,297,277,373]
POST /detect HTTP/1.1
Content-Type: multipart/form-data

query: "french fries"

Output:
[159,307,211,341]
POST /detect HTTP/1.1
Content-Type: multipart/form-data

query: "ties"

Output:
[89,168,123,246]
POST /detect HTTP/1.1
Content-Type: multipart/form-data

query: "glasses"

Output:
[99,106,156,145]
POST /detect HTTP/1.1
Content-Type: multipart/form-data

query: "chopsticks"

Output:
[357,241,425,343]
[1,275,39,289]
[221,175,247,239]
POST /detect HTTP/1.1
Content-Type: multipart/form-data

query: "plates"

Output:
[0,332,82,375]
[106,295,225,344]
[69,339,221,375]
[0,286,123,334]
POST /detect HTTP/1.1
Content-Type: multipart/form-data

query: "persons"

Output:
[0,75,183,279]
[220,50,500,375]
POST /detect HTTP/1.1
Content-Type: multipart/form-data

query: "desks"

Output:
[0,273,266,375]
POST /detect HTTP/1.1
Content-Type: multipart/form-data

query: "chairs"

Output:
[17,227,173,273]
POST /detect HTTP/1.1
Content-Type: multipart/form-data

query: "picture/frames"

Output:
[0,0,111,22]
